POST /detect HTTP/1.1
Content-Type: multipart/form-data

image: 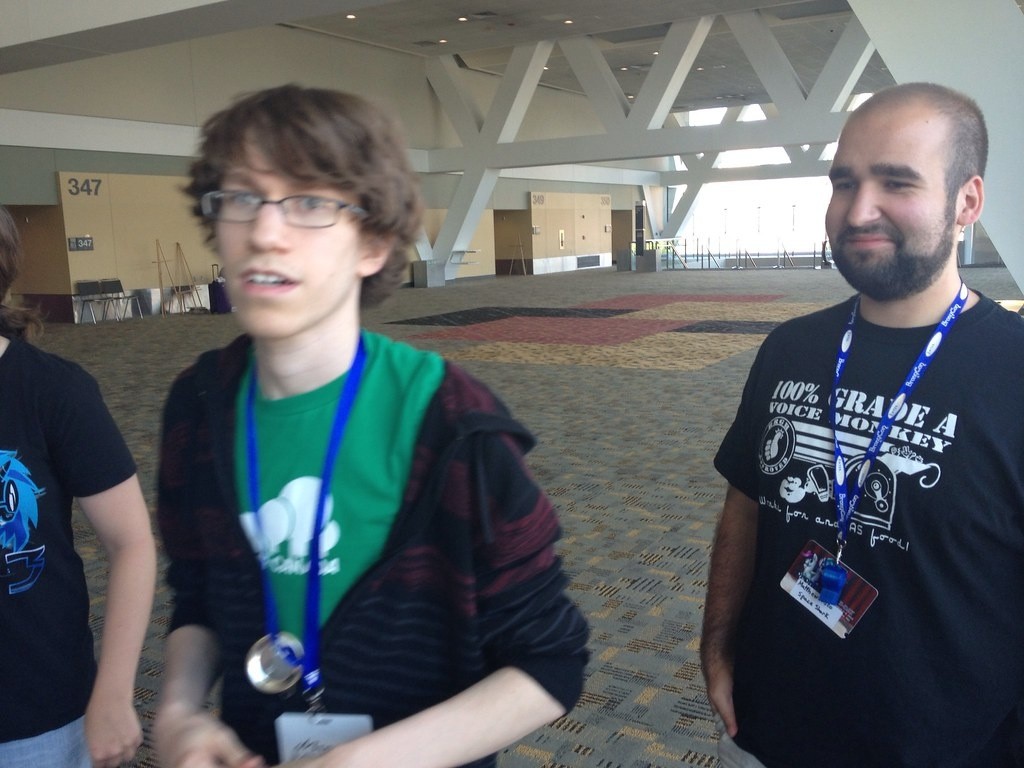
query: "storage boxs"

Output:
[413,258,446,289]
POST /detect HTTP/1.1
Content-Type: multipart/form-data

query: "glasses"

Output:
[202,189,371,229]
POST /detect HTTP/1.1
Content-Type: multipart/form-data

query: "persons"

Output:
[693,82,1024,767]
[0,206,161,768]
[145,81,592,768]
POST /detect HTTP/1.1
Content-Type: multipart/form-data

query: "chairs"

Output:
[100,278,144,322]
[76,279,120,324]
